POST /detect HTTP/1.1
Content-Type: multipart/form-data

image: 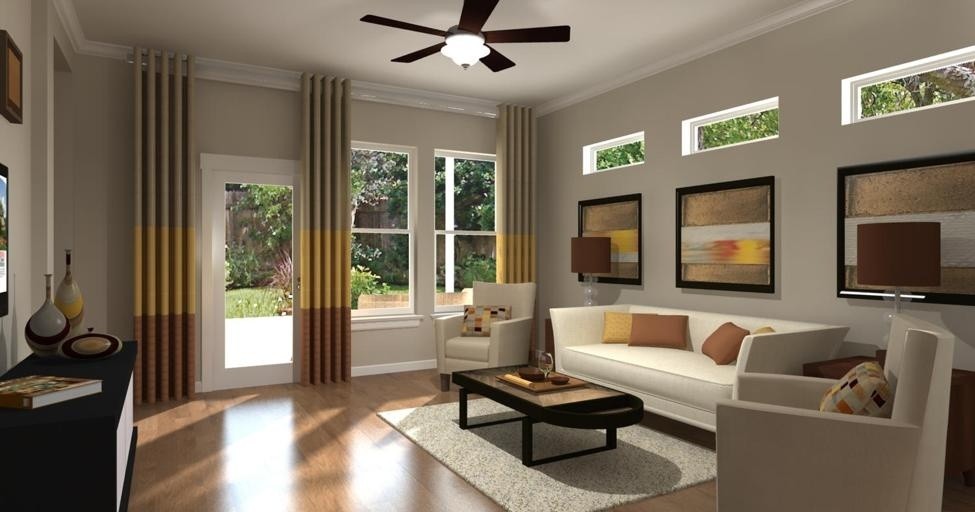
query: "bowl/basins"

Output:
[519,367,549,381]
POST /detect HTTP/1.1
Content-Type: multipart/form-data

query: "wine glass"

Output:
[538,352,554,383]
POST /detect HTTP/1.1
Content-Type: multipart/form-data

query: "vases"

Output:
[21,242,126,367]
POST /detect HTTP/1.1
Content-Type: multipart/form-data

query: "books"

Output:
[0,373,104,410]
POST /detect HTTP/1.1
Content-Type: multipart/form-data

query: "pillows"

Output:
[601,309,690,348]
[457,298,511,338]
[820,358,894,417]
[701,318,773,364]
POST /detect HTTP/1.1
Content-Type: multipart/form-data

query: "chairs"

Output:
[713,312,960,512]
[432,273,537,392]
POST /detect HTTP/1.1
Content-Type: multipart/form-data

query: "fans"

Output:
[360,0,573,78]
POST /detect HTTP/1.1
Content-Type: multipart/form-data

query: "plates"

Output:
[549,377,569,384]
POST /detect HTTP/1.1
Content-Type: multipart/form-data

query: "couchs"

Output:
[546,299,852,434]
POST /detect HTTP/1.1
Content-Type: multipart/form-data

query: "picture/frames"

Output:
[835,151,975,310]
[673,173,778,296]
[0,25,25,127]
[575,191,644,285]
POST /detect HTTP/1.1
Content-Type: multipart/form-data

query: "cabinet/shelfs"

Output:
[1,338,140,508]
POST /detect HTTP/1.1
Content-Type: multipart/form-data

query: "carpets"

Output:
[377,398,722,510]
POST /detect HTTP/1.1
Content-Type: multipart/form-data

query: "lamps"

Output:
[855,219,944,309]
[569,234,614,306]
[435,29,493,74]
